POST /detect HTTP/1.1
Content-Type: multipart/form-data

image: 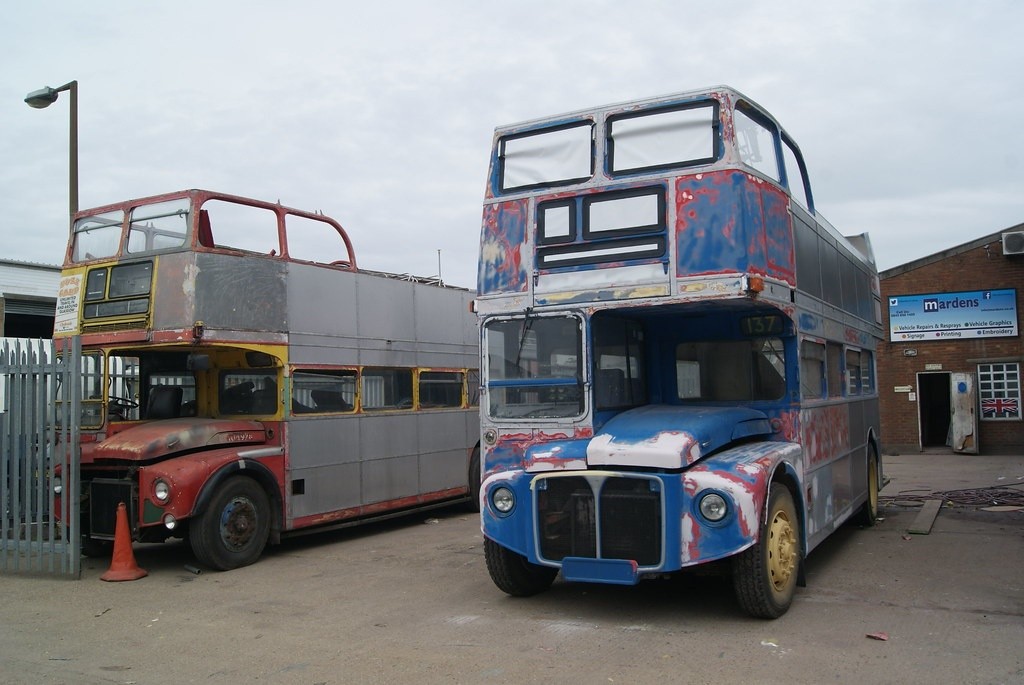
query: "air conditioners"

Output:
[1002,231,1024,255]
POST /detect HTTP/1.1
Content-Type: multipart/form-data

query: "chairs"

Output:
[137,378,348,419]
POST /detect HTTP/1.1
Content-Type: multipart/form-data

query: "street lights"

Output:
[21,78,79,261]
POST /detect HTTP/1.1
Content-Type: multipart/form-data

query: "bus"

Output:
[466,82,888,618]
[46,186,543,569]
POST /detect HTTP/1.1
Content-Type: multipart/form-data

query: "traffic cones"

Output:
[101,498,148,582]
